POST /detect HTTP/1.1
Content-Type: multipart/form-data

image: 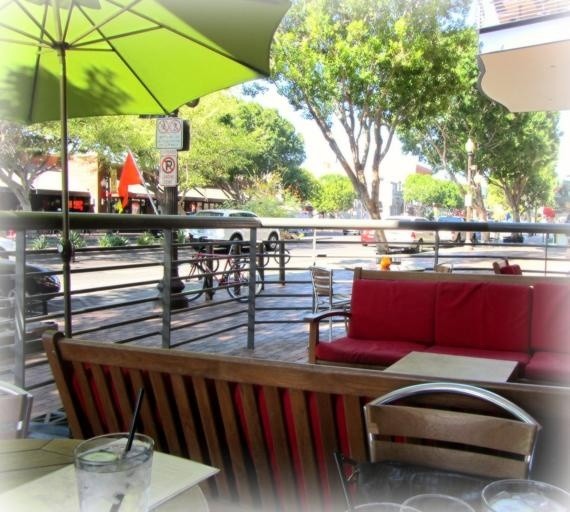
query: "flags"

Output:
[117,153,143,209]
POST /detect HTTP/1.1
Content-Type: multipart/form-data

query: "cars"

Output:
[0,249,63,313]
[381,214,440,253]
[361,230,376,246]
[437,216,479,244]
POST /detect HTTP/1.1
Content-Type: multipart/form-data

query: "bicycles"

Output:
[176,242,264,302]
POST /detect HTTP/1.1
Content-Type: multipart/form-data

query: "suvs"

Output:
[182,208,281,251]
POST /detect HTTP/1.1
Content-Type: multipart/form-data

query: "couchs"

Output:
[305,267,570,386]
[42,329,570,512]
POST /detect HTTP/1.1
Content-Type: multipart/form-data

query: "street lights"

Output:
[464,137,475,246]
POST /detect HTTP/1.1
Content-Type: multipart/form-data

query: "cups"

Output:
[74,432,155,512]
[481,478,570,512]
[369,258,377,269]
[345,503,420,512]
[400,493,477,512]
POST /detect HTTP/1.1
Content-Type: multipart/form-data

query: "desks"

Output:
[346,262,423,272]
[382,350,518,382]
[0,437,220,512]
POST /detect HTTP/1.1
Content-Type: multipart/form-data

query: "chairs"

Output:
[333,382,541,512]
[0,381,33,439]
[309,267,352,342]
[493,257,521,275]
[436,262,453,272]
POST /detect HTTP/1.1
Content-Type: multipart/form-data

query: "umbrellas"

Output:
[0,1,294,338]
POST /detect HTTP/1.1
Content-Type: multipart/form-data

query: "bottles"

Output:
[380,255,391,269]
